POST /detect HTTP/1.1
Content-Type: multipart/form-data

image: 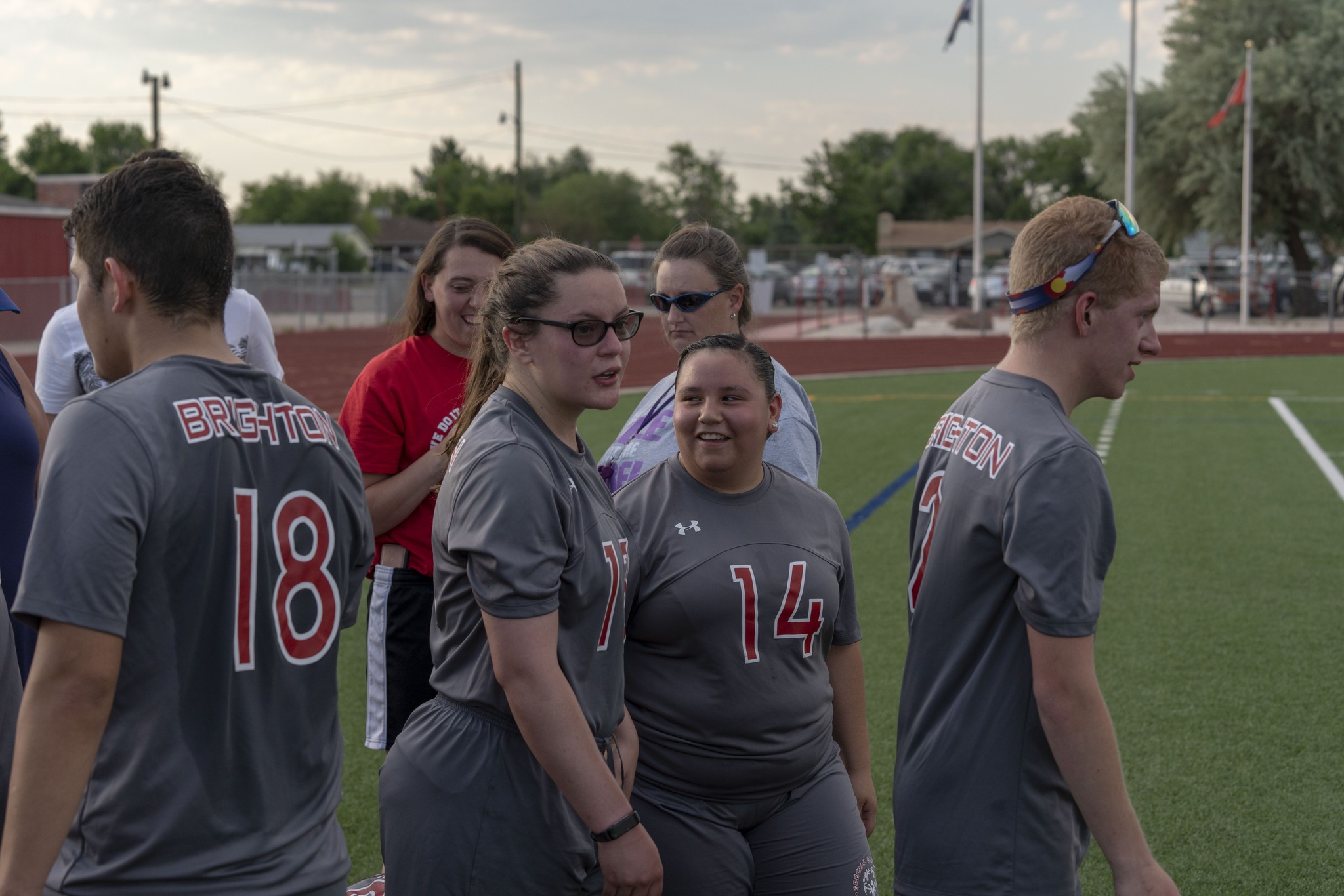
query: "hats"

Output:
[0,286,21,314]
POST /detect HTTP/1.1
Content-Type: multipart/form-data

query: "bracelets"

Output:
[590,809,641,842]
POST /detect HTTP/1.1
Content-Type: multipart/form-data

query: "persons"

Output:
[37,144,285,429]
[0,289,50,836]
[378,234,663,896]
[891,193,1178,896]
[593,222,823,497]
[327,215,516,896]
[609,333,878,896]
[0,159,374,896]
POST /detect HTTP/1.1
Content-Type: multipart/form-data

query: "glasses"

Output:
[515,308,644,346]
[650,288,728,312]
[1105,199,1140,239]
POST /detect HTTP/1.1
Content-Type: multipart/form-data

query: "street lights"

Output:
[142,67,172,150]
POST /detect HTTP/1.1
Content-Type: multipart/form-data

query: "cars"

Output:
[1200,251,1344,323]
[608,250,658,295]
[744,252,1013,315]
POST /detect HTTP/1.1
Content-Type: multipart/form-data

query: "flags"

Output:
[942,0,971,51]
[1207,68,1245,128]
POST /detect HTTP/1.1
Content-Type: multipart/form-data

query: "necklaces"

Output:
[598,382,675,481]
